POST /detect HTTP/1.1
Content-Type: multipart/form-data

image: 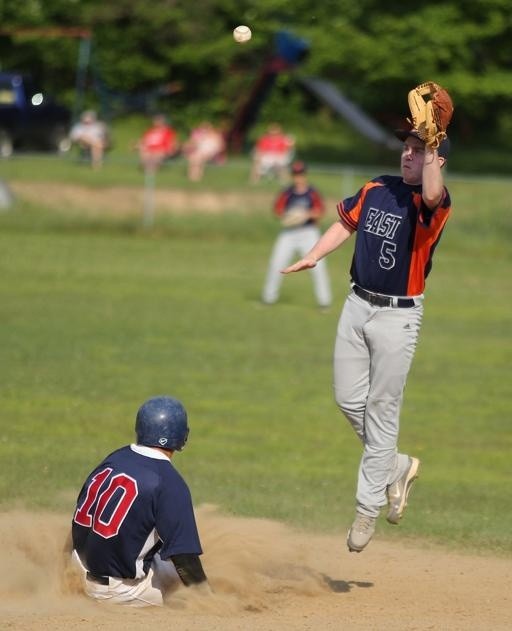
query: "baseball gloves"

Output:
[406,80,454,148]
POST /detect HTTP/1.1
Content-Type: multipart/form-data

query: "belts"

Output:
[348,281,415,309]
[85,570,111,587]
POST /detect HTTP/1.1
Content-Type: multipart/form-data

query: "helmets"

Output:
[134,396,188,453]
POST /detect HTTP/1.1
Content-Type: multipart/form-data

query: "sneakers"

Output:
[346,512,379,550]
[381,456,420,524]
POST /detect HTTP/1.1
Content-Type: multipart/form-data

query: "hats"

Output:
[291,161,307,173]
[396,122,453,159]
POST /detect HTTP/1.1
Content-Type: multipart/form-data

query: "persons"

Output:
[131,113,180,179]
[262,159,334,310]
[67,109,114,175]
[180,121,227,184]
[245,122,296,189]
[278,81,455,558]
[61,393,219,609]
